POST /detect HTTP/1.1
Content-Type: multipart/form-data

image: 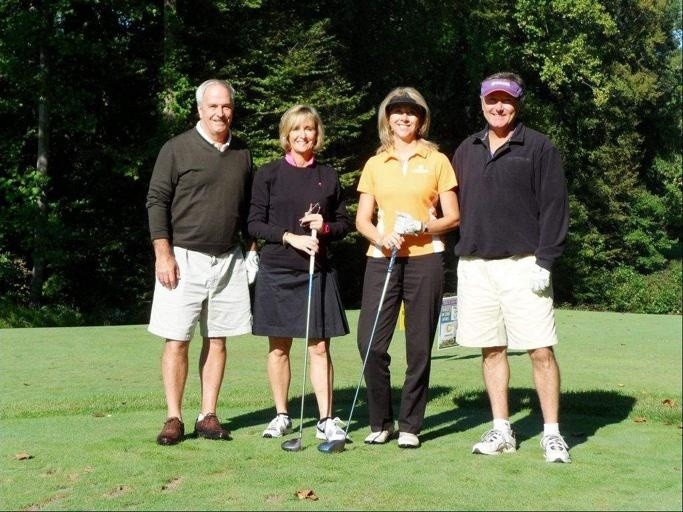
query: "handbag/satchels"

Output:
[423,225,428,236]
[282,232,288,246]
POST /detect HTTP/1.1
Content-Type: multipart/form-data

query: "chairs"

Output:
[363,430,391,445]
[470,429,518,457]
[315,417,349,443]
[540,433,572,464]
[396,430,420,448]
[262,415,294,440]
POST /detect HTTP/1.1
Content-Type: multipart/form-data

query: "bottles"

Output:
[479,79,525,99]
[384,95,425,111]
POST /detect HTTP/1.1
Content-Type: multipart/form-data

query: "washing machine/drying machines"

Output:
[318,235,402,452]
[281,203,320,451]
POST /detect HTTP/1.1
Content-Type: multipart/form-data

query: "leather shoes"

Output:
[531,265,550,296]
[395,211,424,237]
[243,250,260,286]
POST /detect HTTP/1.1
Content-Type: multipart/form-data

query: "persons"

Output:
[247,105,352,444]
[354,86,461,448]
[145,79,259,445]
[452,71,570,463]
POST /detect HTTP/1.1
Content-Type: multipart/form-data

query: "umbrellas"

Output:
[194,413,228,441]
[156,417,185,446]
